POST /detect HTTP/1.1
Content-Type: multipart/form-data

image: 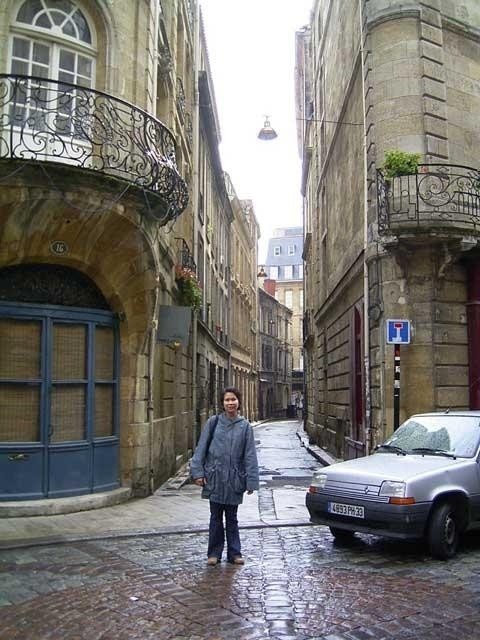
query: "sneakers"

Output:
[229,554,244,564]
[208,557,221,565]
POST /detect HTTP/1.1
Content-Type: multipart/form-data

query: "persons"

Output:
[189,387,259,565]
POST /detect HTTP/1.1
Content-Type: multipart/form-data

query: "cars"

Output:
[306,410,480,560]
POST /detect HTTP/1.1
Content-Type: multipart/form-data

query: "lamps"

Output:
[257,114,364,141]
